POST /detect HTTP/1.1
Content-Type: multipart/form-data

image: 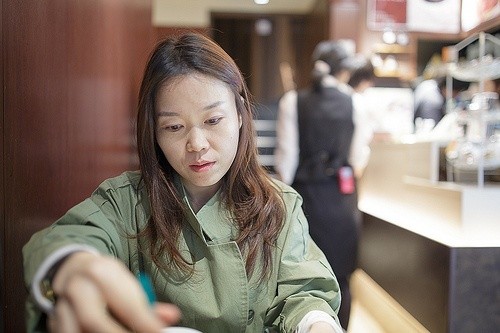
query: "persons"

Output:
[273,41,370,330]
[22,32,348,333]
[415,72,468,129]
[350,58,372,92]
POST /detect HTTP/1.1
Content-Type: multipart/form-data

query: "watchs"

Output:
[41,260,60,306]
